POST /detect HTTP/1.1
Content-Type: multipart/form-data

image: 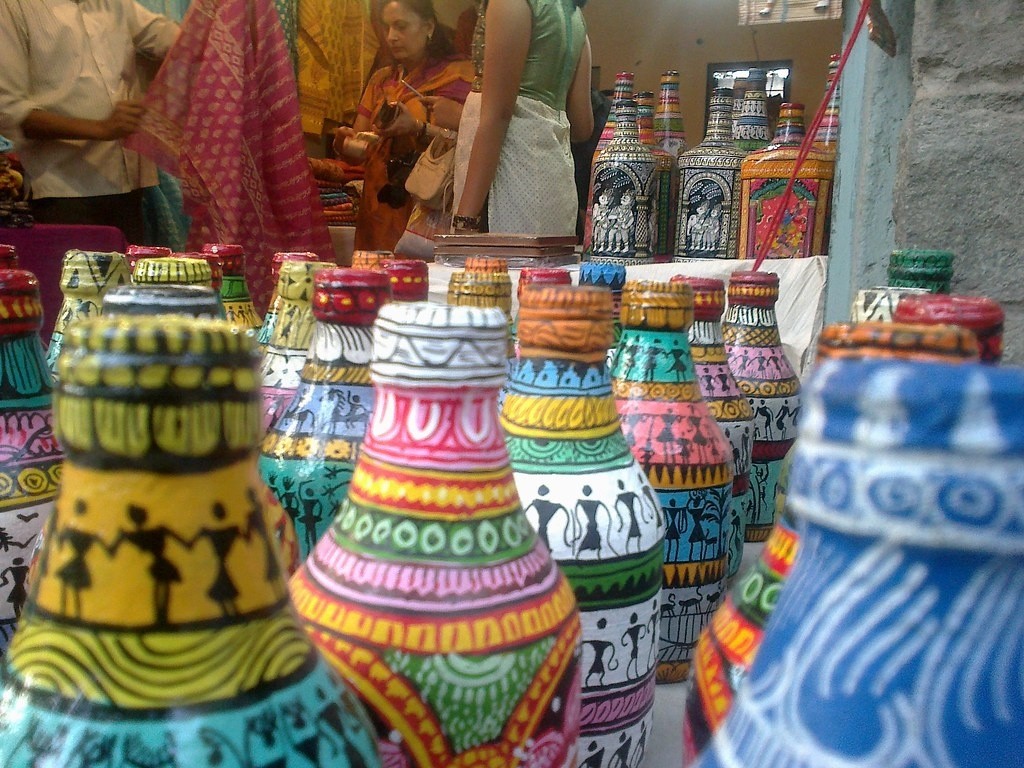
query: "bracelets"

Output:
[333,140,340,155]
[417,122,430,146]
[452,215,480,231]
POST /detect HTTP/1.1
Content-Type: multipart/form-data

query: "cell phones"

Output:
[400,79,423,98]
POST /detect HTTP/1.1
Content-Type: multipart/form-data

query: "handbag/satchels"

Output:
[394,200,454,262]
[404,136,455,211]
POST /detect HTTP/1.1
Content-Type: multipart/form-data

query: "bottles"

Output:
[0,54,1024,768]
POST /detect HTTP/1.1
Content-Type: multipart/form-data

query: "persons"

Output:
[450,0,595,235]
[331,0,476,252]
[417,97,464,131]
[0,1,183,245]
